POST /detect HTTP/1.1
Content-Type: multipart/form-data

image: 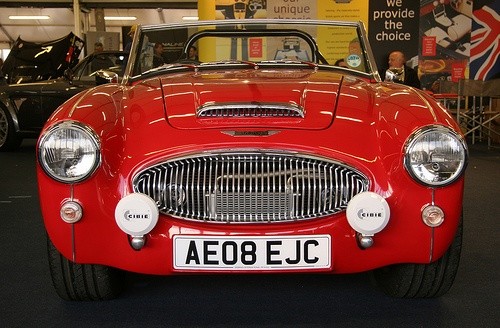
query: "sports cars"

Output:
[35,17,468,302]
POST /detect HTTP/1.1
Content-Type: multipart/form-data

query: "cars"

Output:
[0,51,134,152]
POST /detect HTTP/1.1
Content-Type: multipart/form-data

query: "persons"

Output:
[189,46,198,61]
[381,51,420,89]
[334,38,367,72]
[153,43,169,68]
[91,42,114,77]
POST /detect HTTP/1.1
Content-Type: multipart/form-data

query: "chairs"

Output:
[439,79,500,150]
